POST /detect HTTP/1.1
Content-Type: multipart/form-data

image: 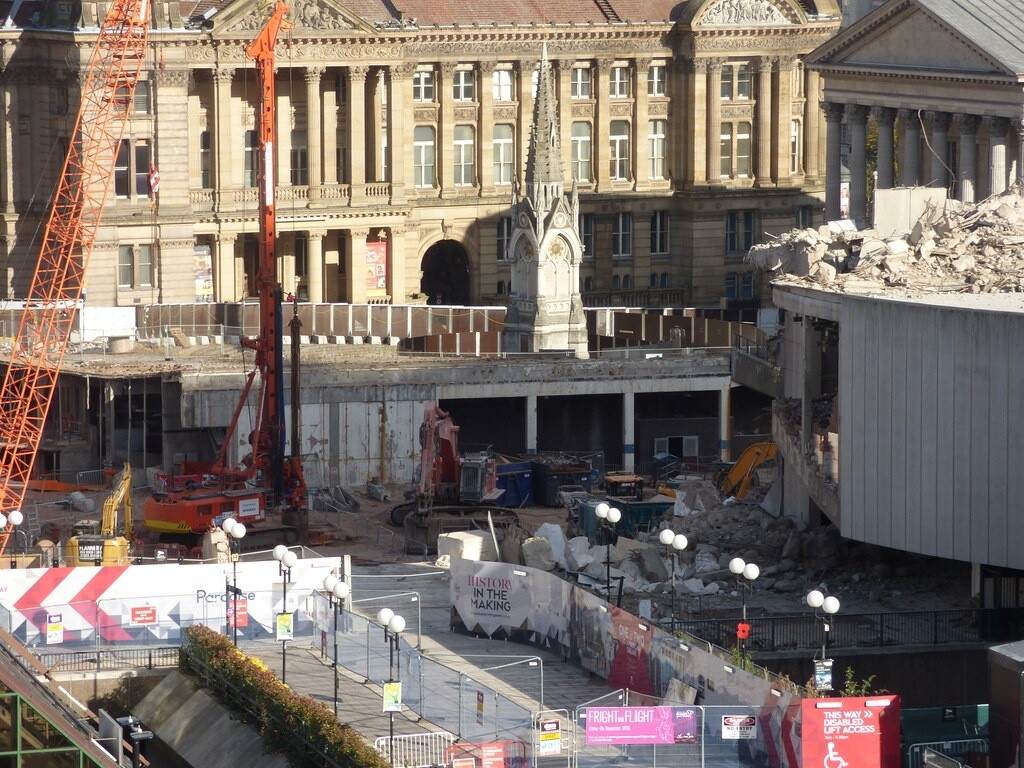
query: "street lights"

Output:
[377,607,406,763]
[324,576,348,717]
[660,529,688,635]
[595,502,621,603]
[273,544,298,685]
[0,512,23,569]
[807,590,841,698]
[729,557,760,669]
[222,519,246,647]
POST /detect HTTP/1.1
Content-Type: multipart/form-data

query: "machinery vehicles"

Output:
[143,0,308,555]
[386,400,520,535]
[656,441,778,506]
[66,461,140,566]
